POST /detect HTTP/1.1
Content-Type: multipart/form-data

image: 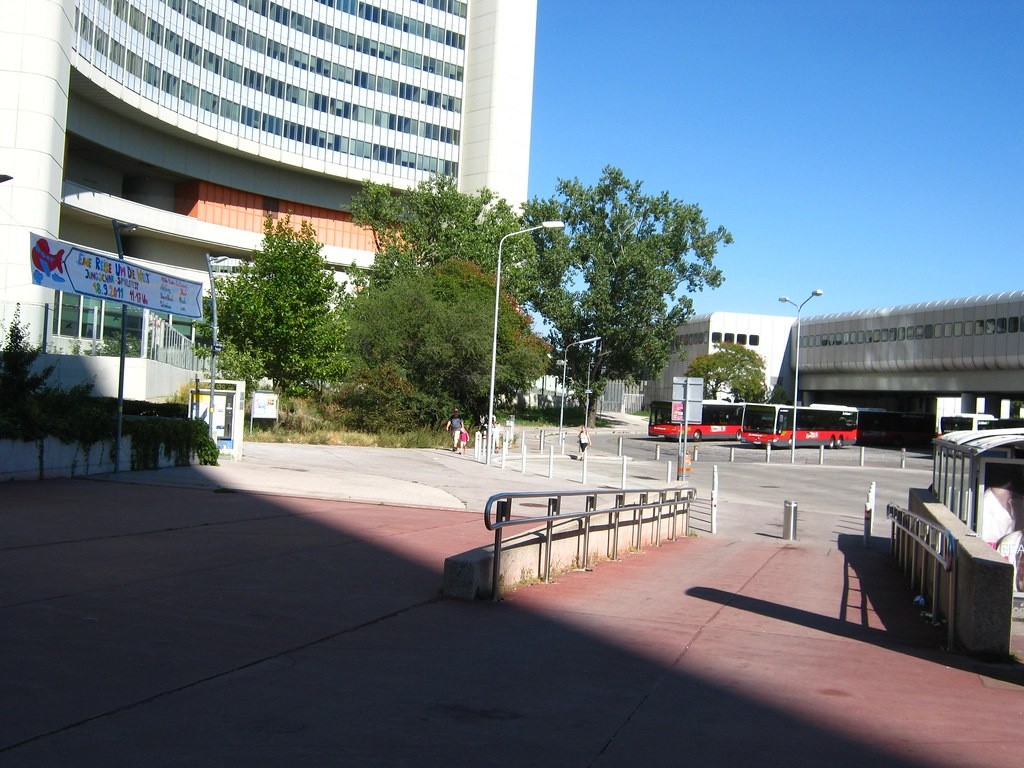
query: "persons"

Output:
[578,426,592,460]
[459,428,469,455]
[446,408,463,451]
[480,415,499,453]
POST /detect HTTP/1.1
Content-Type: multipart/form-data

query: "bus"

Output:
[812,404,936,447]
[941,414,999,438]
[741,401,859,449]
[641,398,755,442]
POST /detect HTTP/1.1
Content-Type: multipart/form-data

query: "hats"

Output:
[453,409,459,413]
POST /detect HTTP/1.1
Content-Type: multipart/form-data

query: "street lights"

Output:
[110,219,140,473]
[779,289,823,463]
[560,337,602,450]
[486,221,565,465]
[205,254,228,439]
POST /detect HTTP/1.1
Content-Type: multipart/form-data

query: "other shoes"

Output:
[453,447,457,451]
[459,452,462,455]
